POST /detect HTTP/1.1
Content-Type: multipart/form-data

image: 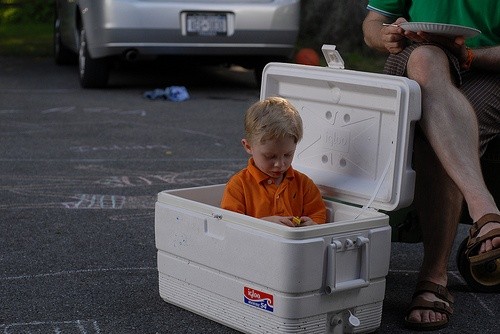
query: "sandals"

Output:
[403,271,456,329]
[461,213,500,264]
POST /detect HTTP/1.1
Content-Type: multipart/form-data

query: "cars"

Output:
[55,0,300,91]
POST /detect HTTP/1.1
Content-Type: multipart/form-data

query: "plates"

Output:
[397,22,482,40]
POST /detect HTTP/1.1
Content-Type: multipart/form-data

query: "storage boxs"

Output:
[154,62,421,334]
